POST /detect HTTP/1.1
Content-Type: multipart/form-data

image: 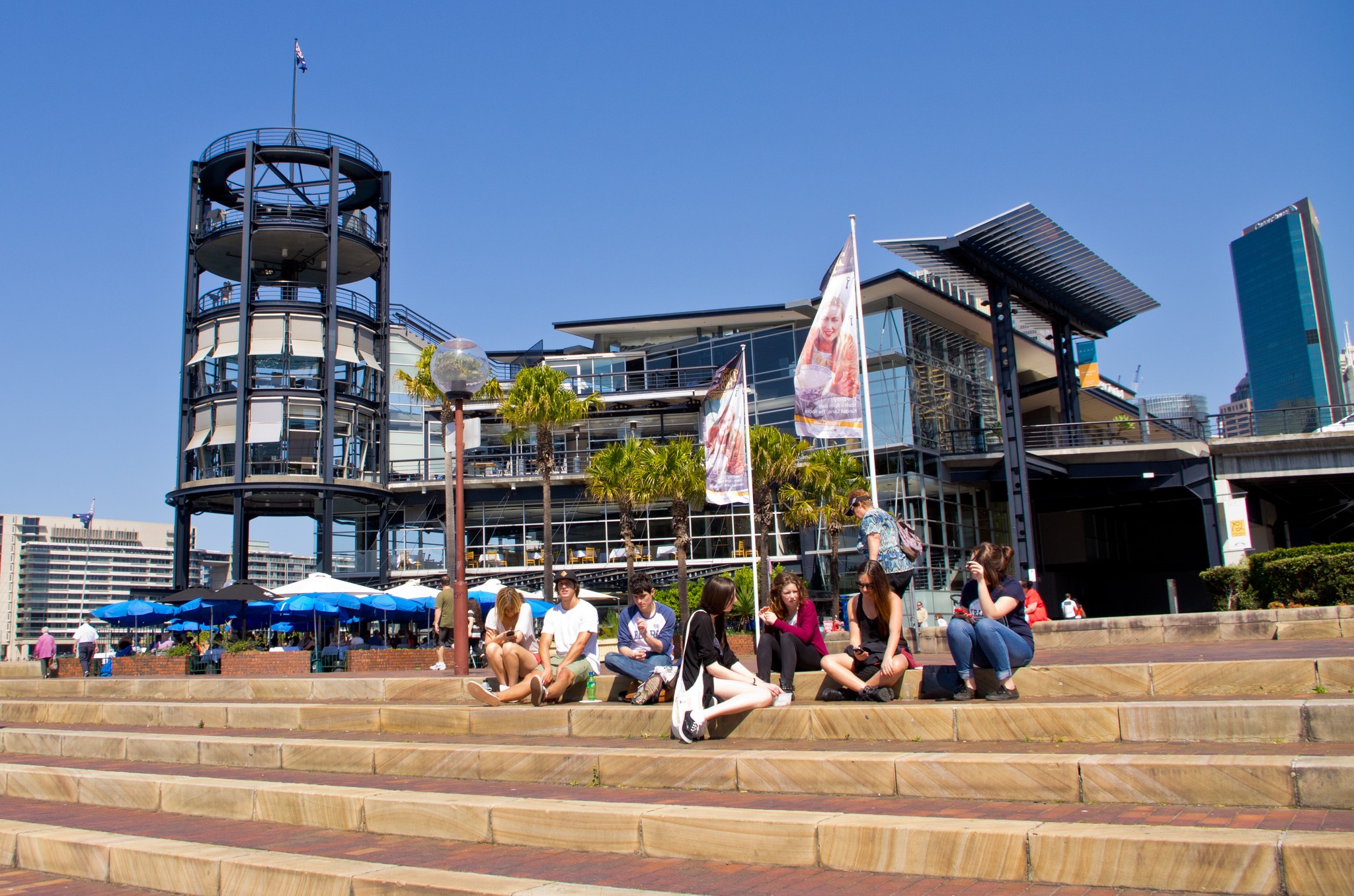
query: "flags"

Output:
[701,350,749,504]
[295,42,307,73]
[794,232,864,439]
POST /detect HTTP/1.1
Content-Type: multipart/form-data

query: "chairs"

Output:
[523,547,600,566]
[253,457,271,474]
[509,554,521,566]
[465,550,509,568]
[554,458,567,474]
[731,537,759,558]
[388,552,395,570]
[675,552,686,560]
[498,462,510,477]
[633,545,643,562]
[300,457,312,474]
[398,551,425,570]
[267,372,282,388]
[644,546,655,561]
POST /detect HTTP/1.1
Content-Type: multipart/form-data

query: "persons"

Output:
[1062,593,1082,619]
[820,560,923,702]
[34,627,56,678]
[913,602,928,627]
[756,572,829,700]
[843,489,914,654]
[219,282,228,304]
[468,597,488,668]
[430,576,454,670]
[946,542,1034,700]
[935,613,947,626]
[73,617,435,677]
[484,586,541,703]
[467,569,601,707]
[707,405,745,476]
[1019,579,1048,627]
[679,576,787,743]
[605,571,678,705]
[799,296,858,397]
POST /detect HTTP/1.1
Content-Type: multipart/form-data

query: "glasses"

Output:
[855,579,875,589]
[850,505,854,515]
[917,604,921,606]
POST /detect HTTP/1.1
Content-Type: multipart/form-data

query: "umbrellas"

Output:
[90,572,507,659]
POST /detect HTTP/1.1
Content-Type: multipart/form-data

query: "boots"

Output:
[822,687,858,701]
[856,685,896,702]
[778,677,795,701]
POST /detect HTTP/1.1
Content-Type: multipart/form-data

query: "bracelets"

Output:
[753,678,757,686]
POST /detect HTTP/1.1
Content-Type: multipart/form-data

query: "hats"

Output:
[844,496,872,517]
[553,570,578,586]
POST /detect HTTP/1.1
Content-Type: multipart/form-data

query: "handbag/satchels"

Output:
[920,621,928,628]
[49,655,58,671]
[886,511,922,562]
[671,675,706,739]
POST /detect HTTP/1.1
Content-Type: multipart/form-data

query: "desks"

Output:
[479,554,504,567]
[390,555,416,570]
[656,546,677,560]
[296,379,312,389]
[712,544,731,558]
[528,552,554,565]
[572,550,597,563]
[471,463,499,477]
[609,548,652,562]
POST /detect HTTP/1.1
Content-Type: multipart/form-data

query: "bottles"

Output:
[587,671,596,701]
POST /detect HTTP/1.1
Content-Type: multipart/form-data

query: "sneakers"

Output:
[632,676,661,704]
[650,665,678,687]
[679,710,701,744]
[985,685,1020,700]
[705,695,718,709]
[530,675,549,706]
[430,662,447,670]
[952,685,980,701]
[467,680,500,706]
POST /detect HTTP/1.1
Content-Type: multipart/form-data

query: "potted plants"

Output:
[986,421,1004,454]
[1103,414,1136,444]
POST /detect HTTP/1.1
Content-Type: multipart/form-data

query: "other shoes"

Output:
[481,655,488,668]
[43,673,49,679]
[84,672,90,677]
[508,699,520,704]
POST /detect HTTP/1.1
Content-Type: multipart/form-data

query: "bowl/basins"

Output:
[794,364,835,400]
[706,453,728,480]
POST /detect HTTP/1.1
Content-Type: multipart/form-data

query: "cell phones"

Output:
[954,609,972,618]
[852,648,865,654]
[506,631,515,636]
